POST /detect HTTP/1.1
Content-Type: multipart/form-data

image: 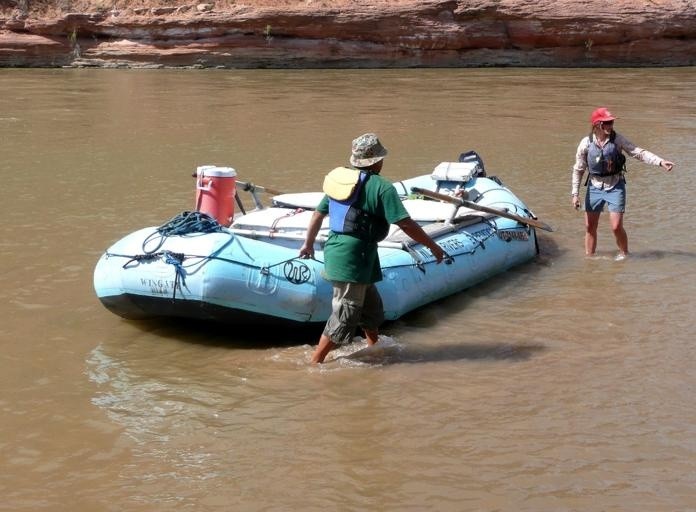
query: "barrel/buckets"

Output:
[196,165,236,228]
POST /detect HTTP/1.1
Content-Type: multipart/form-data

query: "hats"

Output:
[350,134,389,168]
[591,107,615,125]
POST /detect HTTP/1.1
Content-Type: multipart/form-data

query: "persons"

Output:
[572,108,676,263]
[298,132,444,365]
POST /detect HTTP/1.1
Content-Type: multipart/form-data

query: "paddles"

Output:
[411,187,553,232]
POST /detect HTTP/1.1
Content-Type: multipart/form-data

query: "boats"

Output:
[93,150,540,327]
